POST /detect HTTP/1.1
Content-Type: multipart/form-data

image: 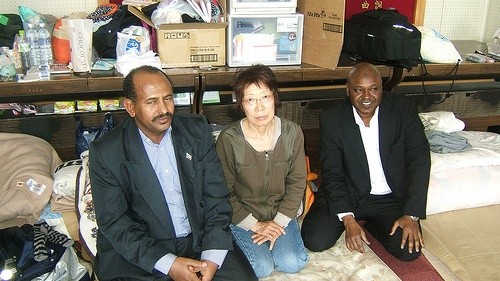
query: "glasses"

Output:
[240,93,275,105]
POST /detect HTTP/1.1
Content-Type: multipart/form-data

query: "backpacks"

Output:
[341,8,421,96]
[411,24,463,104]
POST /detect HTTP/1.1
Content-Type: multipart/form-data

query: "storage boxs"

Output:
[127,0,228,68]
[227,12,304,66]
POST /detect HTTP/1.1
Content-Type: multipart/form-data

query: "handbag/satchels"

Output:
[0,4,151,76]
[75,112,115,159]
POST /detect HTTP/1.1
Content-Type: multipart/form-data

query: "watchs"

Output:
[410,216,419,220]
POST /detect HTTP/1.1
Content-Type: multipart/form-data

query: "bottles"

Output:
[18,23,53,70]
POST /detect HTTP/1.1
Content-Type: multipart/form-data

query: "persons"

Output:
[89,65,258,281]
[215,65,309,278]
[300,62,431,262]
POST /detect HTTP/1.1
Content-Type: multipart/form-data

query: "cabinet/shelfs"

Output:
[0,41,500,162]
